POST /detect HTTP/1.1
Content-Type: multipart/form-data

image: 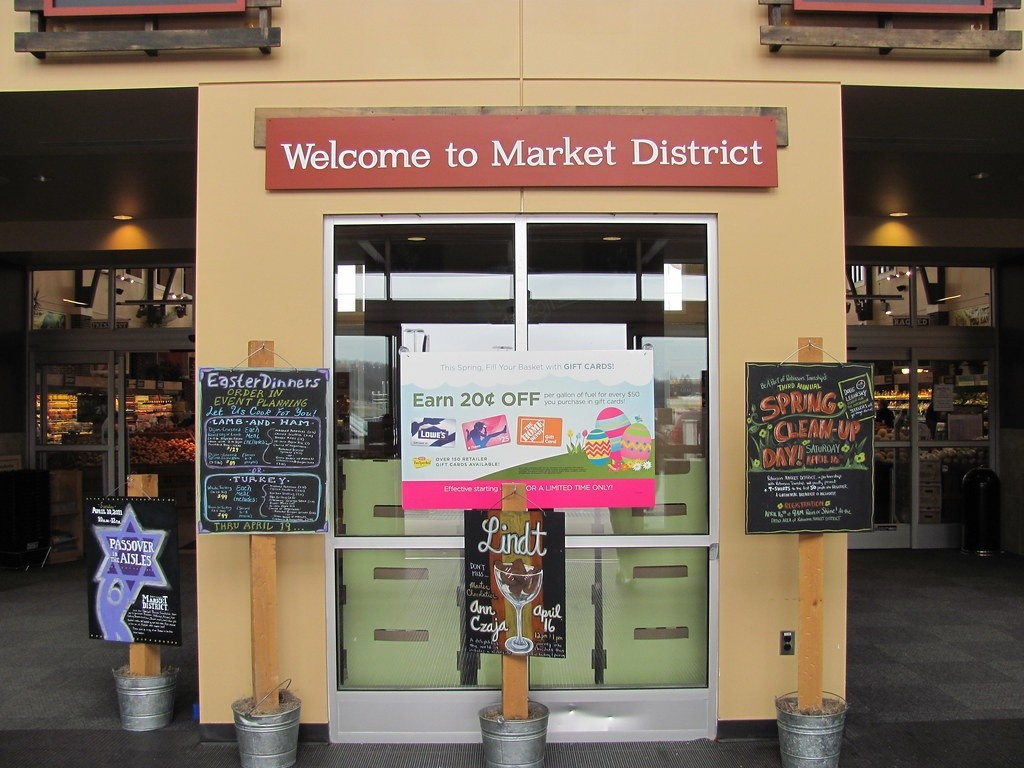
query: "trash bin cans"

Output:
[959,464,1002,556]
[0,469,52,569]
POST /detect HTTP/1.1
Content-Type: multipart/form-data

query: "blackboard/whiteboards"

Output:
[196,366,331,535]
[742,359,876,538]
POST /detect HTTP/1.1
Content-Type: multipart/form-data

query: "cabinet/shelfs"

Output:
[919,460,941,525]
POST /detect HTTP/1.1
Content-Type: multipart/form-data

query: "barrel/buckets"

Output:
[114,666,179,731]
[231,695,301,768]
[777,690,845,768]
[479,701,549,768]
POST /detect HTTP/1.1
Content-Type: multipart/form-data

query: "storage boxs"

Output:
[71,313,91,328]
